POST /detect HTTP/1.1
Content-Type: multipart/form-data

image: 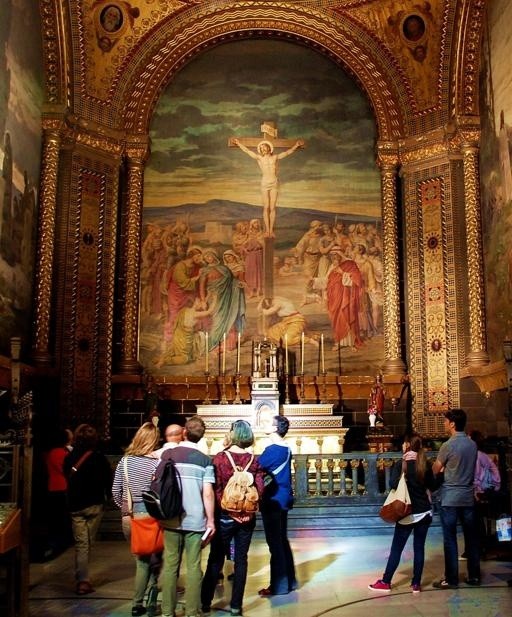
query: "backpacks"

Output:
[142,459,185,527]
[221,451,259,512]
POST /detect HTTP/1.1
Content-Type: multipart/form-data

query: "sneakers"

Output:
[132,570,271,617]
[369,553,481,592]
[75,584,93,595]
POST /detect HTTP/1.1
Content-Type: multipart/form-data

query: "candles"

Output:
[301,332,305,372]
[237,331,240,373]
[285,332,289,373]
[321,333,325,372]
[222,332,226,373]
[205,332,209,371]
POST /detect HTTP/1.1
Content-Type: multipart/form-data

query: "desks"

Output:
[0,501,21,617]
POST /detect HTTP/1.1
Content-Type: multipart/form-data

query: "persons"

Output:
[367,435,436,594]
[63,423,113,595]
[144,375,154,396]
[43,430,74,510]
[371,374,386,422]
[432,408,482,587]
[459,430,499,561]
[112,384,300,616]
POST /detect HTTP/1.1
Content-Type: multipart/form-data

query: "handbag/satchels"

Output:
[380,472,412,523]
[130,518,165,555]
